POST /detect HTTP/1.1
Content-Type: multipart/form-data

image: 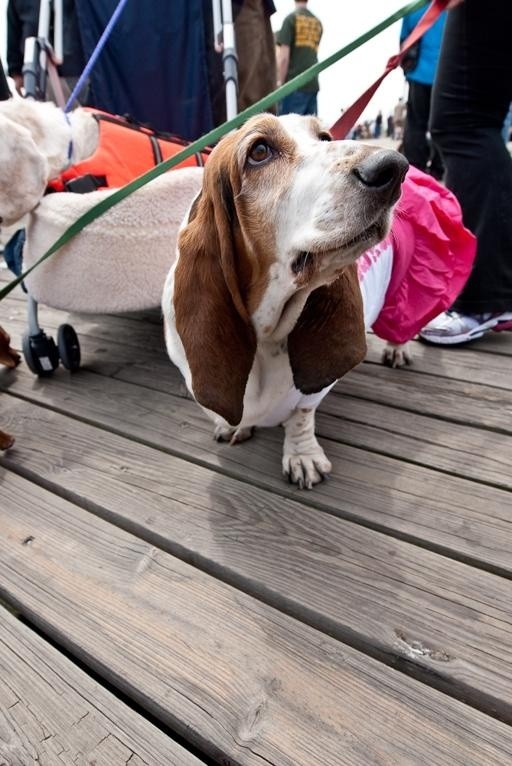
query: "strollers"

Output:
[16,0,239,377]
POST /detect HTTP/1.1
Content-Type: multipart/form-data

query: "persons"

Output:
[352,97,407,141]
[277,0,323,116]
[419,0,512,345]
[400,5,448,181]
[7,0,91,109]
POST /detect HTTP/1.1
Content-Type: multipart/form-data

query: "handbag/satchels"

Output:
[45,105,215,201]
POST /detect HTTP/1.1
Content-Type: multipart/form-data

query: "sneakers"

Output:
[415,306,512,345]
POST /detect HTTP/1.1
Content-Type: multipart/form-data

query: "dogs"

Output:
[0,94,100,450]
[161,113,414,489]
